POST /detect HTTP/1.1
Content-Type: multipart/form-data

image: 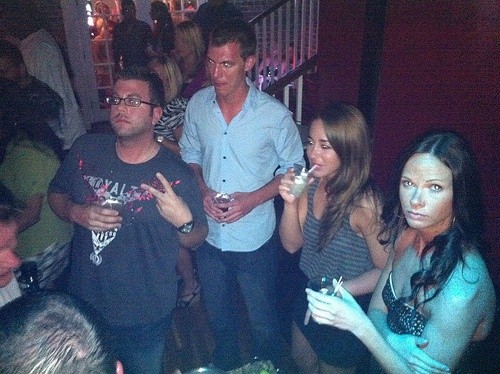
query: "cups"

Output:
[212,190,234,229]
[286,164,314,199]
[307,276,337,300]
[97,194,125,233]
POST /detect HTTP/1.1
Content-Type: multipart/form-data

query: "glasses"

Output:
[104,95,159,107]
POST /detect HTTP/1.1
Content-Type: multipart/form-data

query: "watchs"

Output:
[155,134,164,143]
[179,218,196,235]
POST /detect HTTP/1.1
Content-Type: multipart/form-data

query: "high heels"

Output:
[175,282,202,310]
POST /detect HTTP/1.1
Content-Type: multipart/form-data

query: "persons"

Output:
[145,54,203,308]
[304,130,498,374]
[47,66,209,374]
[178,17,307,374]
[0,0,88,311]
[0,290,126,374]
[278,101,392,374]
[90,0,308,159]
